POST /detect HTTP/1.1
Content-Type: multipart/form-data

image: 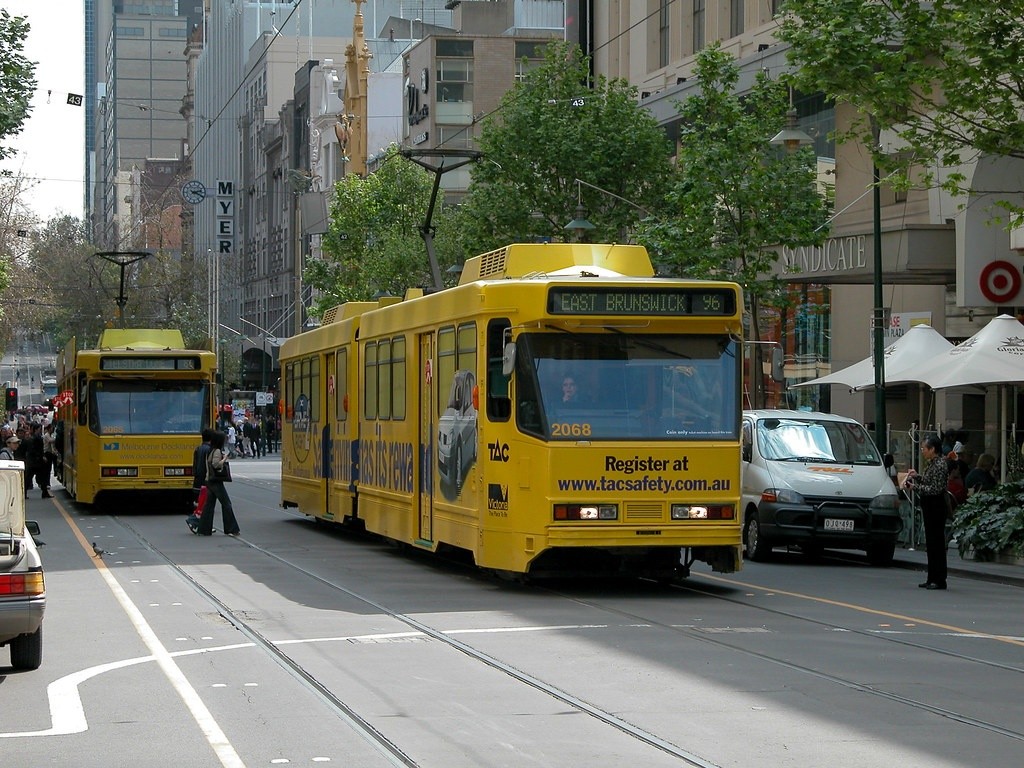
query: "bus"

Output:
[278,148,783,585]
[54,250,232,515]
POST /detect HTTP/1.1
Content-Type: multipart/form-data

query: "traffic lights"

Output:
[6,388,17,411]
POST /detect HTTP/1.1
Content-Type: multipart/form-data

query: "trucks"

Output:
[40,367,57,405]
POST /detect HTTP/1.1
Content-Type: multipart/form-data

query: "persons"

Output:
[198,434,240,537]
[25,423,54,499]
[0,436,22,460]
[949,453,998,500]
[898,434,950,590]
[225,414,282,460]
[554,370,590,409]
[186,429,216,535]
[0,413,65,447]
[54,421,65,483]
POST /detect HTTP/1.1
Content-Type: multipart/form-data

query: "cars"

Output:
[23,404,48,415]
[0,460,47,671]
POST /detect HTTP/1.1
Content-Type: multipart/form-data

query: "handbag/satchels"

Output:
[208,449,232,482]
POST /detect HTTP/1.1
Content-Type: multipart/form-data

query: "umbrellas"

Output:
[785,312,1024,484]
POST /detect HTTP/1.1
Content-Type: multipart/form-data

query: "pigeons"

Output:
[92,542,111,559]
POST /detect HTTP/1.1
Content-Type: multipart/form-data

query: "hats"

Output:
[5,436,22,445]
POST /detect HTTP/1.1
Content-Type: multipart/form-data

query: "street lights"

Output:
[236,318,279,452]
[769,76,887,459]
[214,322,257,390]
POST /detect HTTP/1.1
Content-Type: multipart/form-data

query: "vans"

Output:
[743,410,905,566]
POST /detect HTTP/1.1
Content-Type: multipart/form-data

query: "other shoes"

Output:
[185,519,199,533]
[216,528,217,533]
[228,529,241,536]
[917,579,947,590]
[42,492,53,498]
